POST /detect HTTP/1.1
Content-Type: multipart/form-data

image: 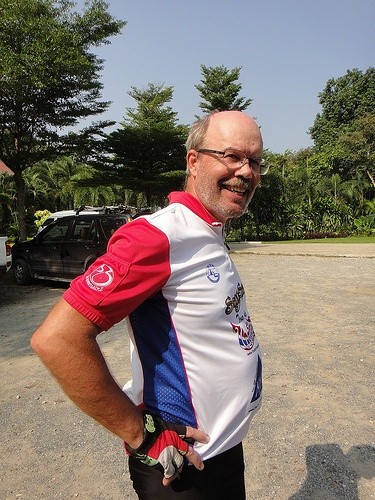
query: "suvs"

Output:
[10,205,153,287]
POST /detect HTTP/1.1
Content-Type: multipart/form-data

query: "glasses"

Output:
[196,148,271,175]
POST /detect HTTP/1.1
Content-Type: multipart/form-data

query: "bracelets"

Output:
[126,411,161,461]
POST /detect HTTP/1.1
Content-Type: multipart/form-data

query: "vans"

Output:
[36,209,101,238]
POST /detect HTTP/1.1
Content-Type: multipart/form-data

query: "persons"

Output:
[30,111,263,500]
